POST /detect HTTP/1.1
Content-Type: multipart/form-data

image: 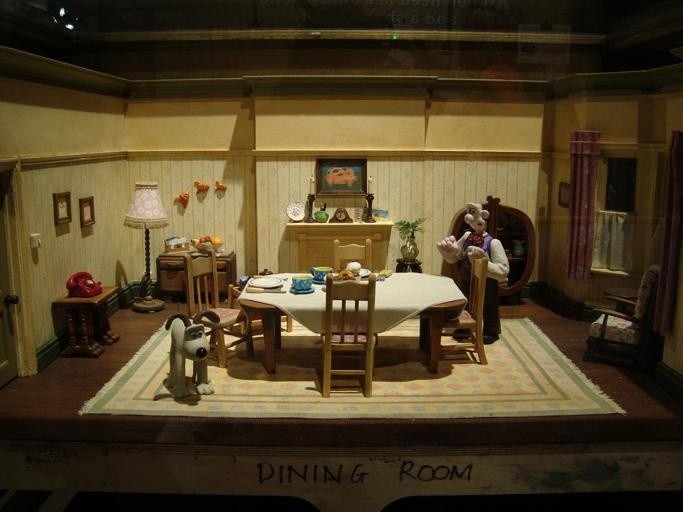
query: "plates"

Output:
[312,278,326,284]
[290,287,314,294]
[248,275,283,289]
[287,202,305,221]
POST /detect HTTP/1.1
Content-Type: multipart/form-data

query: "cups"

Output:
[311,267,333,280]
[292,275,312,290]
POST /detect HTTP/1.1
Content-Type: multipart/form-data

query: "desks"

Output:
[225,283,292,335]
[52,287,119,359]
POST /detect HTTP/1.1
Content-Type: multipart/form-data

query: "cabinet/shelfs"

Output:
[155,251,236,301]
[287,221,394,274]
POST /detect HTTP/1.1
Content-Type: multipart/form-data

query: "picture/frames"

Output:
[314,158,368,196]
[53,191,72,225]
[79,196,98,227]
[557,182,574,211]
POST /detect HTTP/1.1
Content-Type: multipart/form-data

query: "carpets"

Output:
[79,312,625,417]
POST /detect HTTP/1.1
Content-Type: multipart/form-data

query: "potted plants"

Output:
[394,217,426,260]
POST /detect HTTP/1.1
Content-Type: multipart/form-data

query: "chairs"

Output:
[183,250,250,367]
[315,273,373,396]
[413,257,489,373]
[582,266,661,367]
[332,238,375,276]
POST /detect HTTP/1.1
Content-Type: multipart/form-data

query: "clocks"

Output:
[329,208,354,224]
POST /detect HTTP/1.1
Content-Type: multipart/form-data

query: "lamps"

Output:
[123,184,168,312]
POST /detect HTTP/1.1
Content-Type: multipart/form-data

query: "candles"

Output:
[309,174,314,196]
[369,176,373,195]
